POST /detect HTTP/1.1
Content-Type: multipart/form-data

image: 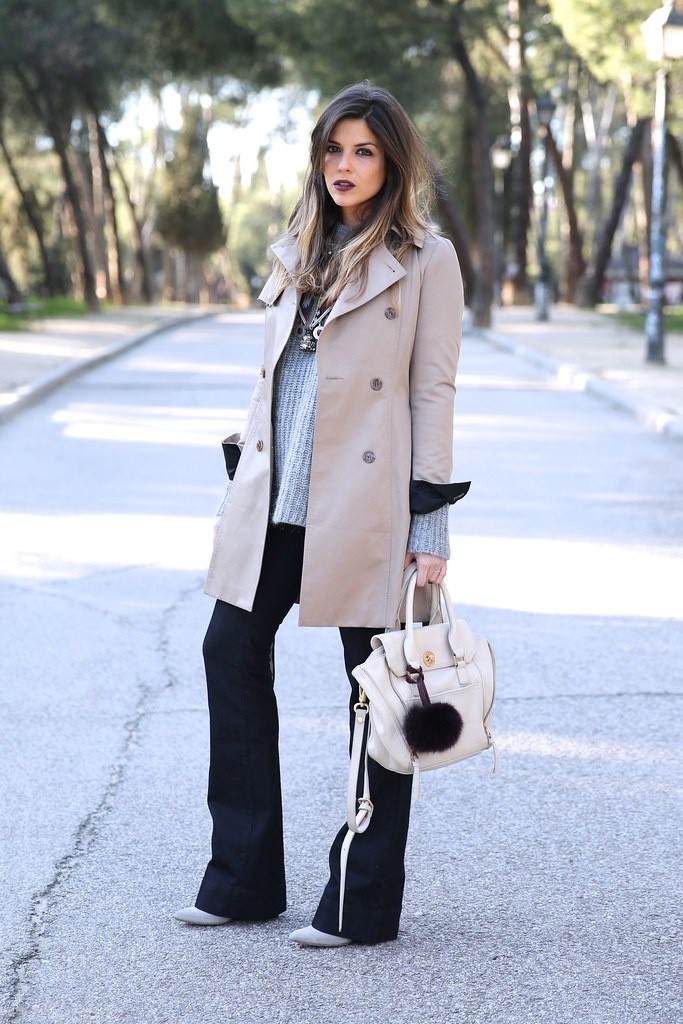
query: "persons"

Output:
[170,85,463,952]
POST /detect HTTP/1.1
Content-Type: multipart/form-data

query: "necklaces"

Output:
[327,241,333,255]
[298,301,337,352]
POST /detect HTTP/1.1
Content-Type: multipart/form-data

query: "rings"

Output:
[435,570,440,572]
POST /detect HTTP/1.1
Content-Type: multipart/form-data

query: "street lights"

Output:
[533,91,557,323]
[640,1,683,363]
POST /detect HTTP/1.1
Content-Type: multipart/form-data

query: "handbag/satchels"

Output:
[337,561,499,773]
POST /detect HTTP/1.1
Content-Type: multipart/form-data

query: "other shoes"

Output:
[288,925,353,947]
[171,906,232,925]
